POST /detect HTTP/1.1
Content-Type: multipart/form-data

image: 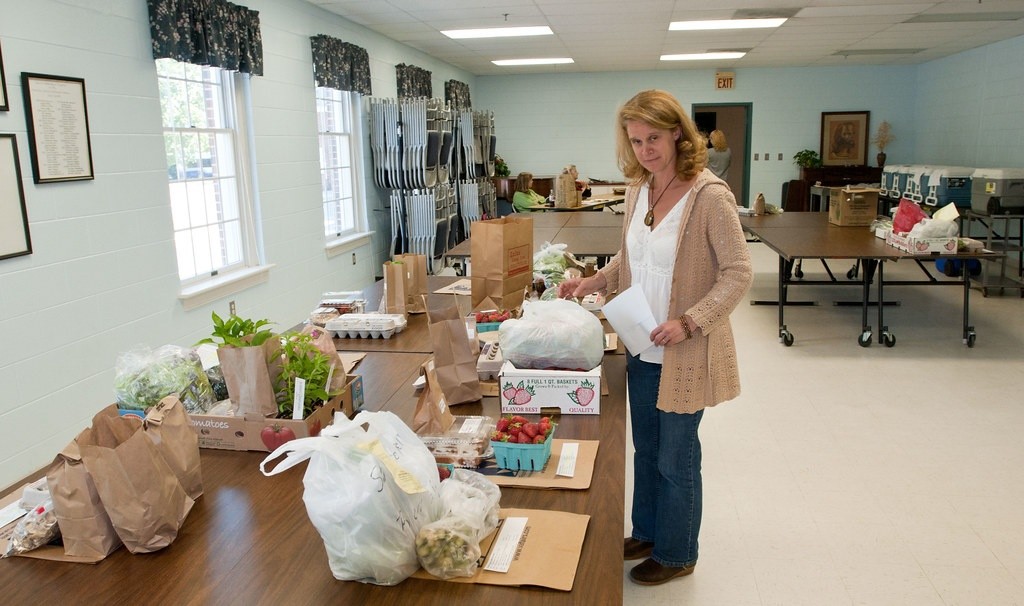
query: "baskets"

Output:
[491,425,555,471]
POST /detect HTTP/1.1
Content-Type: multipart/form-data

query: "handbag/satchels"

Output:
[378,253,429,316]
[470,217,533,313]
[46,394,204,557]
[422,285,482,405]
[499,298,605,371]
[259,410,439,585]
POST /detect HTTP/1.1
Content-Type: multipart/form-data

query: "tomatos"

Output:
[260,422,295,451]
[310,418,322,436]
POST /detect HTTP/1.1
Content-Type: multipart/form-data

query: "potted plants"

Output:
[794,149,818,167]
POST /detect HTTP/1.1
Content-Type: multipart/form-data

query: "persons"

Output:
[558,89,753,586]
[563,164,592,200]
[513,171,548,213]
[700,129,732,182]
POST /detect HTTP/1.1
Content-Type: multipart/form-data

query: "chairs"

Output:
[366,96,516,277]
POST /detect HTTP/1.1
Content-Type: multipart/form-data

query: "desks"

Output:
[0,196,626,606]
[740,211,1024,348]
[809,186,831,212]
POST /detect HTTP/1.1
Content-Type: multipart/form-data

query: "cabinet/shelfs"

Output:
[799,165,881,214]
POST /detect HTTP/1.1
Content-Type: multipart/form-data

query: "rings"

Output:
[663,339,666,344]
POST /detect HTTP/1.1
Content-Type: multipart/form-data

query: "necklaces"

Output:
[631,173,680,255]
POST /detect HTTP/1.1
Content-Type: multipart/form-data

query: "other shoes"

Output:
[622,536,655,560]
[629,559,695,585]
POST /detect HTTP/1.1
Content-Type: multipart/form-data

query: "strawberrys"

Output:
[490,413,555,444]
[475,308,511,322]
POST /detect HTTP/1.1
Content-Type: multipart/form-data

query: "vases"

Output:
[876,153,886,167]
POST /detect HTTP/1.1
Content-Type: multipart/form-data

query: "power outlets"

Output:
[765,153,769,161]
[755,154,759,160]
[778,154,783,161]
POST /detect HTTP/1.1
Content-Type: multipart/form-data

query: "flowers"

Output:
[875,122,896,152]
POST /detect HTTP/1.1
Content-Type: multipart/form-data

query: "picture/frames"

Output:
[0,133,33,261]
[820,110,871,166]
[21,71,95,185]
[0,41,11,113]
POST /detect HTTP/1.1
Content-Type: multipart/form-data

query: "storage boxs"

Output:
[489,420,562,472]
[414,413,493,469]
[112,375,363,453]
[316,300,359,315]
[309,308,341,329]
[323,289,369,313]
[498,354,605,418]
[830,164,1024,255]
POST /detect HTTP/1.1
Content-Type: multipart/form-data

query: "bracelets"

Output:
[680,315,693,341]
